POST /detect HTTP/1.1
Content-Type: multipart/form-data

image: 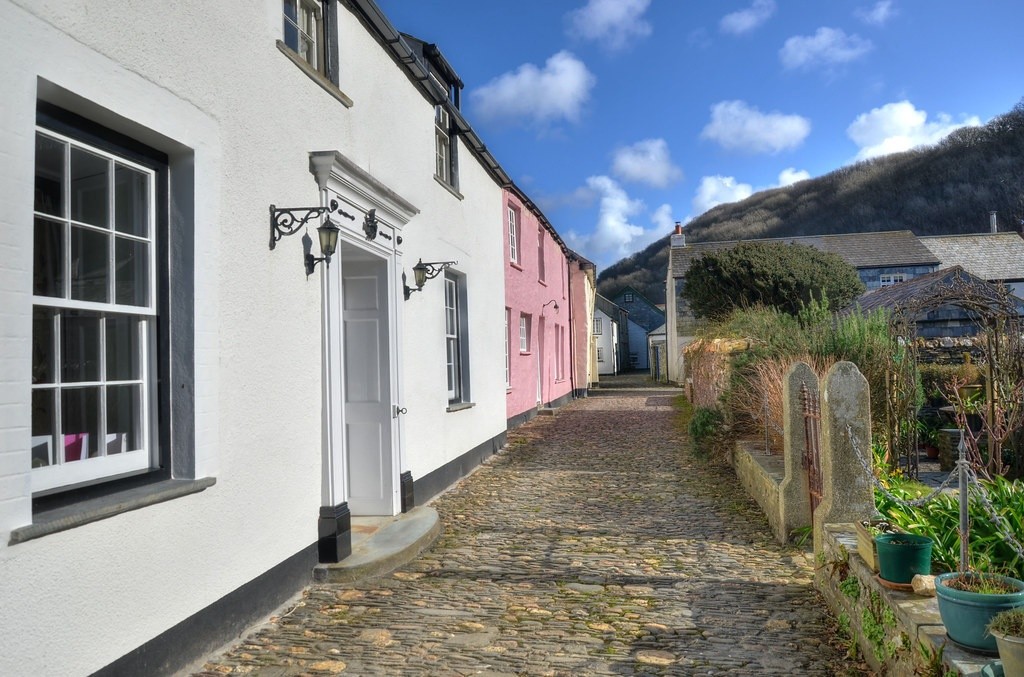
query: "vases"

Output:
[875,533,934,591]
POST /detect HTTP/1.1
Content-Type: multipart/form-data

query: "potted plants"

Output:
[853,518,913,574]
[925,429,940,460]
[918,350,985,407]
[981,607,1024,677]
[955,393,983,431]
[934,528,1024,655]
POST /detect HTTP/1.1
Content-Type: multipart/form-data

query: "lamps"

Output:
[543,300,559,314]
[405,258,428,300]
[305,215,340,277]
